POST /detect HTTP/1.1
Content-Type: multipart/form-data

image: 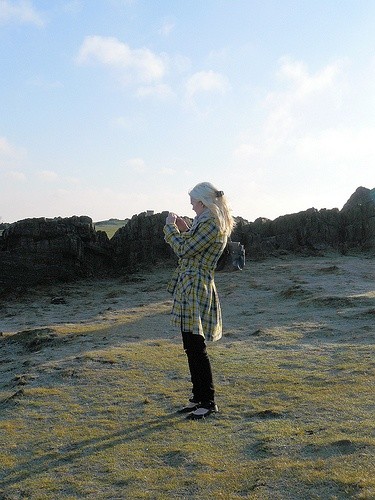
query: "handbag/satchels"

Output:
[214,235,246,274]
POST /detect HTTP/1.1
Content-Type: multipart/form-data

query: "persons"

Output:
[163,182,237,420]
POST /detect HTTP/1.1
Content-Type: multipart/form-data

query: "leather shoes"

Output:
[177,398,219,420]
